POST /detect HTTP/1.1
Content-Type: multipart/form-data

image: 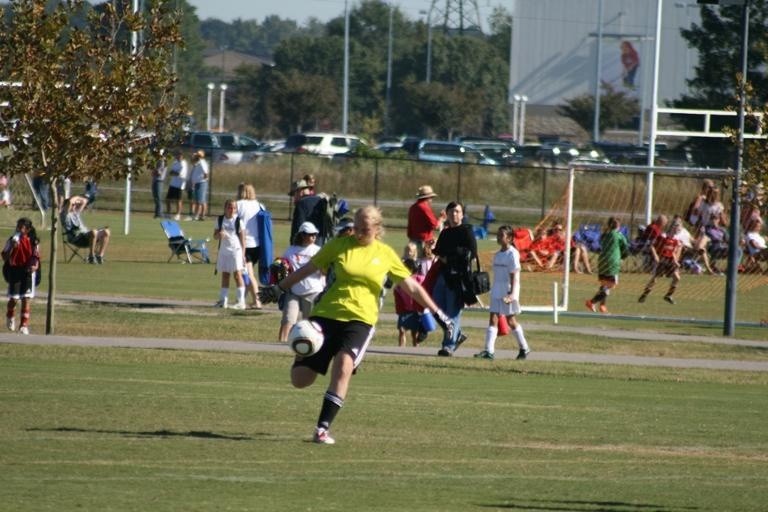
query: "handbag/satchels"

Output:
[472,272,489,294]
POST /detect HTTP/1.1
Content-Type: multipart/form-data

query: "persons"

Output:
[528,177,767,278]
[473,224,531,361]
[59,194,110,263]
[619,41,640,89]
[256,204,456,444]
[0,125,209,220]
[212,171,447,347]
[425,200,479,357]
[584,216,632,315]
[3,218,41,338]
[639,216,683,305]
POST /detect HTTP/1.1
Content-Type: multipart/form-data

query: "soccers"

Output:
[287,321,322,354]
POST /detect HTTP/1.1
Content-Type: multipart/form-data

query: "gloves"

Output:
[257,285,283,305]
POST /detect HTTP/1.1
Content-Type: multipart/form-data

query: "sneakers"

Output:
[584,293,676,313]
[19,327,29,335]
[314,426,335,445]
[215,298,263,311]
[6,319,15,331]
[89,255,104,265]
[439,332,531,360]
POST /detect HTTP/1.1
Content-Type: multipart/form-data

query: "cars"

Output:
[169,131,666,164]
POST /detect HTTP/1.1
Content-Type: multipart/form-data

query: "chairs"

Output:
[159,220,211,265]
[61,220,88,264]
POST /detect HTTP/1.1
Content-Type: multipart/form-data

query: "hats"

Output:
[332,219,354,231]
[415,186,437,200]
[288,179,314,196]
[298,222,319,235]
